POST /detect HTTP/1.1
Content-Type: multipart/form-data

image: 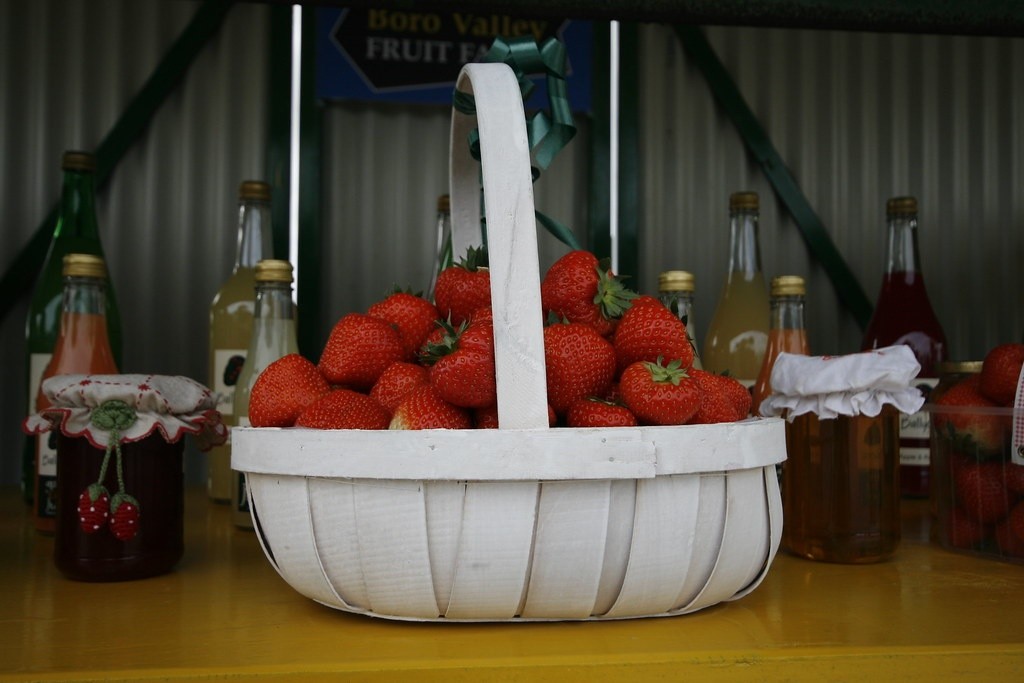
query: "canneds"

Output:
[775,346,902,566]
[42,376,185,581]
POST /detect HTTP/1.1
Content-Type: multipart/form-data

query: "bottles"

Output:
[935,357,983,405]
[858,193,945,498]
[50,417,186,580]
[32,252,117,533]
[17,149,122,507]
[749,272,810,416]
[658,268,700,372]
[230,257,300,525]
[425,193,451,304]
[206,180,277,501]
[777,403,901,562]
[703,191,773,386]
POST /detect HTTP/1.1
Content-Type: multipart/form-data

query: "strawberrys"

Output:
[250,252,753,431]
[933,345,1024,556]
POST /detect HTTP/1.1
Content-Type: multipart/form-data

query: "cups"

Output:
[924,401,1024,566]
